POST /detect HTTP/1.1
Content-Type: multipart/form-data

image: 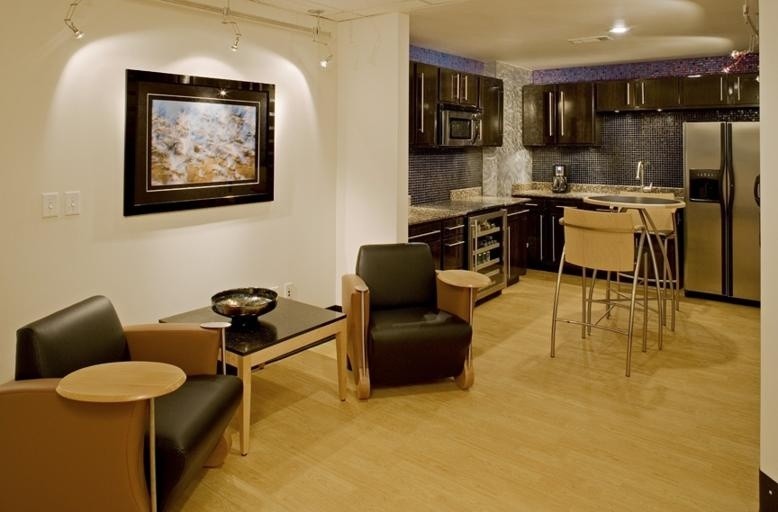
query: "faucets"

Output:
[636,161,652,192]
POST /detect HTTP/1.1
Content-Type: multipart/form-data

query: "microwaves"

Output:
[436,103,483,147]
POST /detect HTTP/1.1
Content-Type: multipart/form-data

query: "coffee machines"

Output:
[552,164,568,193]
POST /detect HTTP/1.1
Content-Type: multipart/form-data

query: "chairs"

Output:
[341,243,494,399]
[0,294,243,512]
[618,191,679,325]
[550,206,649,378]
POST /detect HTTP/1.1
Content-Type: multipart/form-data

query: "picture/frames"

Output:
[123,69,274,215]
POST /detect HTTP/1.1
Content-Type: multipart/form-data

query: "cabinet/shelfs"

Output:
[512,196,684,289]
[408,204,530,303]
[522,81,604,147]
[595,77,680,112]
[438,67,480,108]
[409,60,440,149]
[480,75,504,147]
[681,73,759,108]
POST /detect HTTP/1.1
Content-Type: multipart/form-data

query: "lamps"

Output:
[65,0,86,39]
[221,17,246,52]
[311,39,335,68]
[609,20,630,34]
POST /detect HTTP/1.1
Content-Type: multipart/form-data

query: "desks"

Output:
[582,194,687,350]
[158,296,347,457]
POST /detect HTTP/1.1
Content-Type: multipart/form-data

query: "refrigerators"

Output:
[682,121,760,302]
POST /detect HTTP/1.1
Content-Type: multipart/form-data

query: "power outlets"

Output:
[284,283,298,301]
